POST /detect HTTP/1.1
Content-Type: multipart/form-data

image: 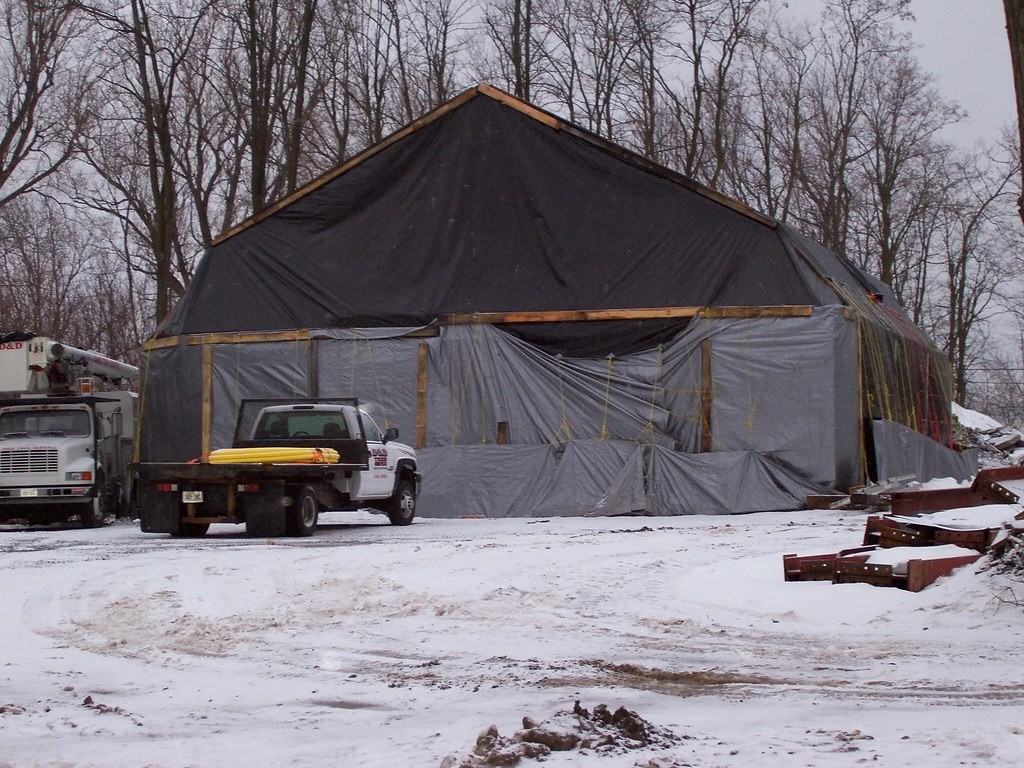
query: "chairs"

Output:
[270,421,289,439]
[323,423,341,439]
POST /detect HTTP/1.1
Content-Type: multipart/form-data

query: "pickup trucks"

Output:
[127,397,424,537]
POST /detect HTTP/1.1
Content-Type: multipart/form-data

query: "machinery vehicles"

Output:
[0,329,143,530]
